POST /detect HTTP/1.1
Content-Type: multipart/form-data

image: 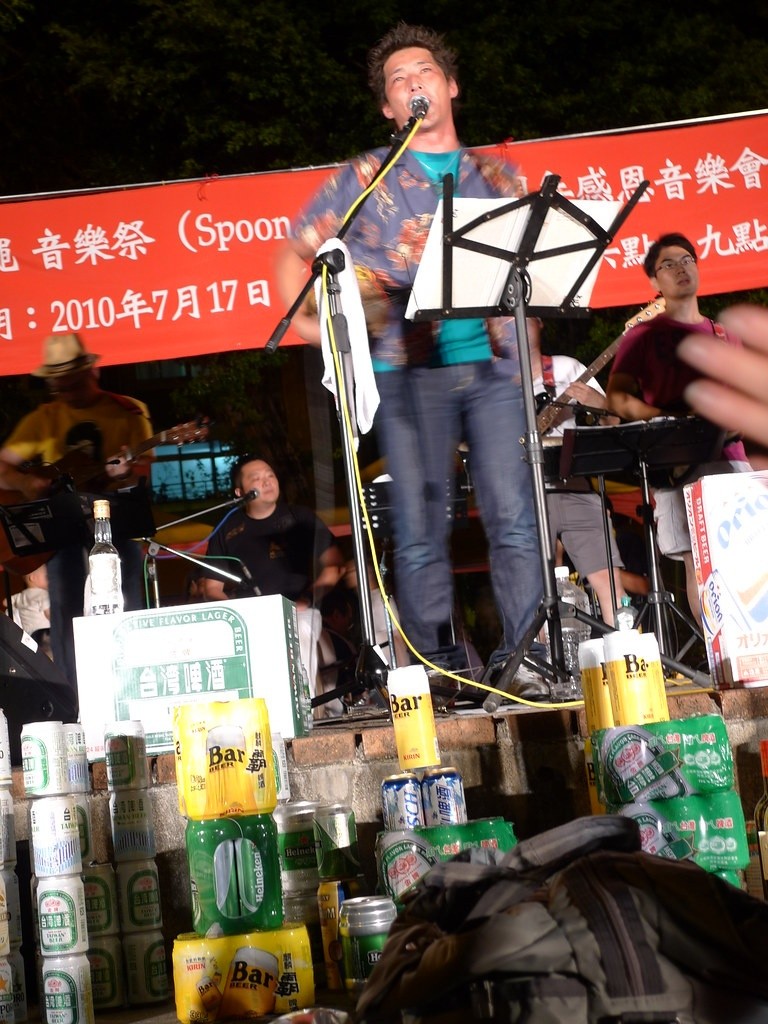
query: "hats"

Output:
[32,333,98,377]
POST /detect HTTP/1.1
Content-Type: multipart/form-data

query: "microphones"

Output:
[408,94,431,119]
[238,488,259,511]
[239,563,262,596]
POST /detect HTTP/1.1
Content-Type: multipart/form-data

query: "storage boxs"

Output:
[72,592,314,763]
[683,469,768,689]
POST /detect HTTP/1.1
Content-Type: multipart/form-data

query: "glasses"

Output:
[654,257,695,275]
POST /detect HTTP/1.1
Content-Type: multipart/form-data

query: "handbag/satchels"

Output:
[344,814,767,1024]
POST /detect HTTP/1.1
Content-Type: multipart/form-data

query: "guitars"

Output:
[0,412,211,576]
[534,293,666,431]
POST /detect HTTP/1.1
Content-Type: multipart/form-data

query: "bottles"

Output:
[754,740,768,900]
[543,566,591,703]
[88,500,124,616]
[616,597,641,631]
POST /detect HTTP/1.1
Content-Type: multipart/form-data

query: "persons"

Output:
[491,305,636,662]
[3,558,50,660]
[275,21,557,699]
[611,232,757,637]
[0,332,157,690]
[197,451,345,716]
[678,302,768,472]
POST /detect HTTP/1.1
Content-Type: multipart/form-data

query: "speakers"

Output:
[0,609,79,766]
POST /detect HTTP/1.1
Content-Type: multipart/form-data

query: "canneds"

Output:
[192,663,520,936]
[197,946,280,1024]
[0,708,170,1024]
[576,627,750,889]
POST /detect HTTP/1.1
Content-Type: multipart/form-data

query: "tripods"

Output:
[260,115,713,715]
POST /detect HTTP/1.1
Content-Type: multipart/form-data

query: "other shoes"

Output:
[429,675,457,708]
[508,664,551,701]
